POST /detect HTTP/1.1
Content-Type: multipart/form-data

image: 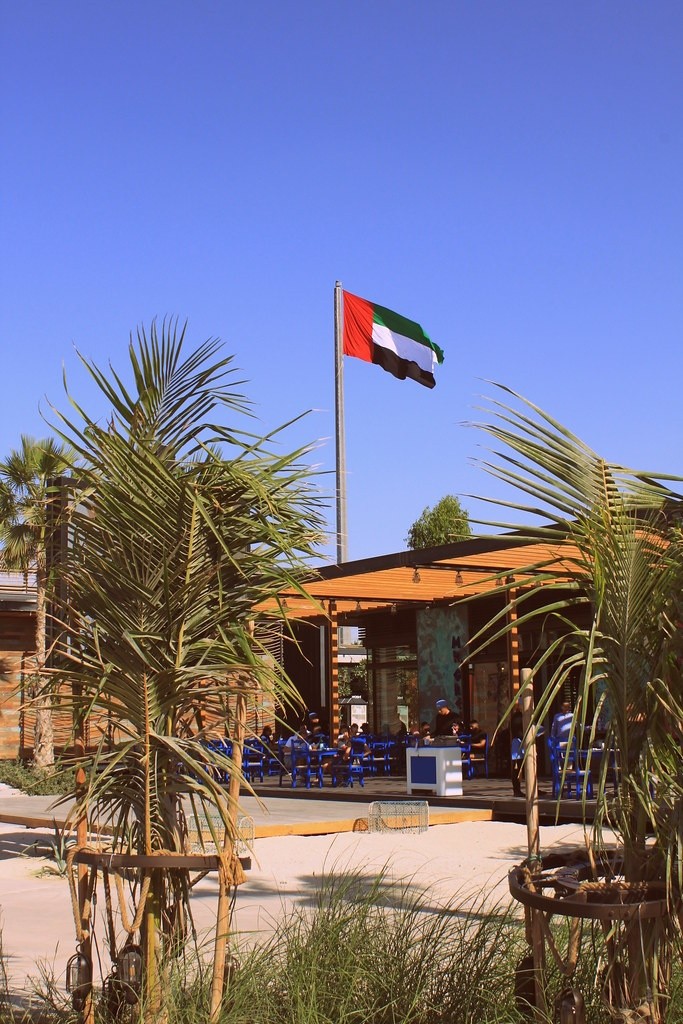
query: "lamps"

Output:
[455,569,463,587]
[534,580,541,588]
[495,572,503,588]
[411,568,421,584]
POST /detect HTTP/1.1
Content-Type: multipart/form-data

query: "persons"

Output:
[260,713,328,780]
[551,702,590,769]
[413,700,487,758]
[512,697,546,796]
[337,724,372,774]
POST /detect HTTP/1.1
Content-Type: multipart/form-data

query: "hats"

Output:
[435,700,447,708]
[309,712,318,718]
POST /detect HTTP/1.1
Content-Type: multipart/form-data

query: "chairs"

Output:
[194,733,619,801]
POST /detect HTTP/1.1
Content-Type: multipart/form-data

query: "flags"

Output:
[342,289,445,389]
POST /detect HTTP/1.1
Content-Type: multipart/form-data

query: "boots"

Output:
[538,790,547,795]
[512,777,526,796]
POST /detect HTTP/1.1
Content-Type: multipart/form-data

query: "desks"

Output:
[561,746,621,798]
[284,747,338,788]
